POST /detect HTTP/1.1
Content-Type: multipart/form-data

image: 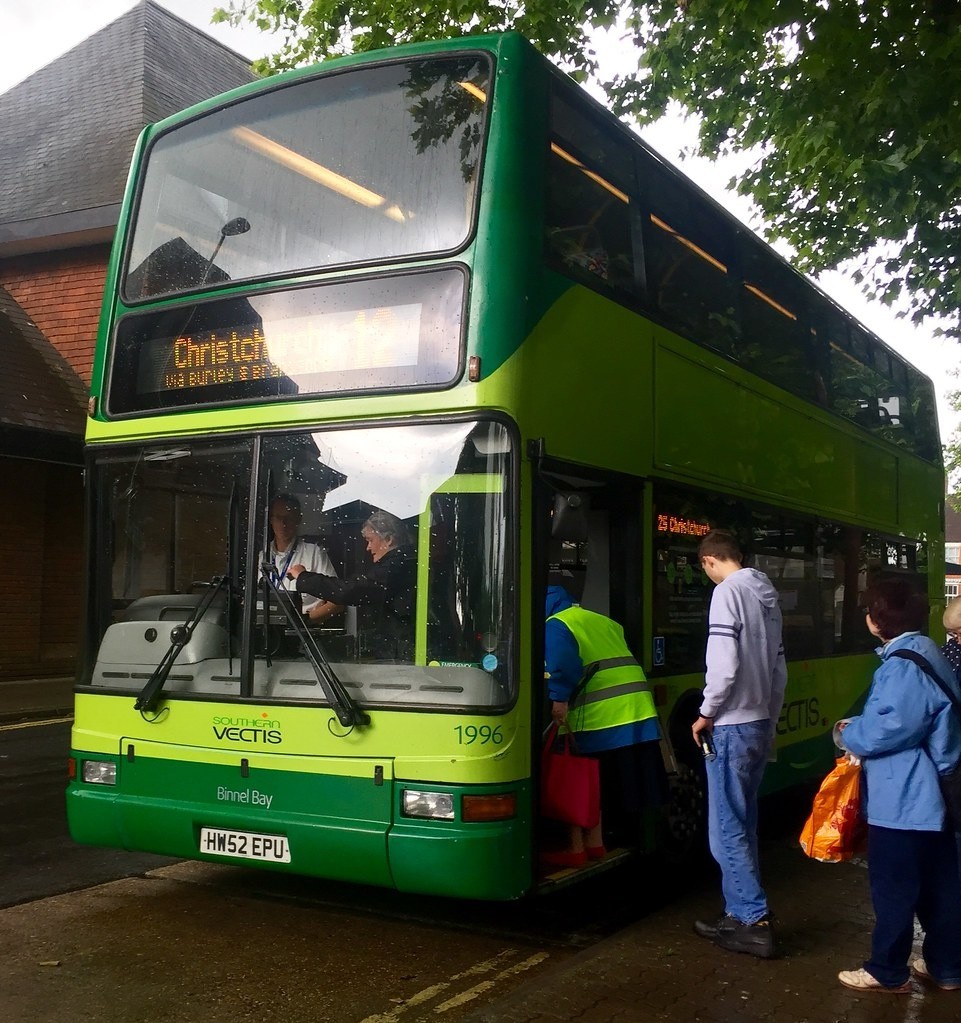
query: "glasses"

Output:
[946,629,961,638]
[861,607,871,620]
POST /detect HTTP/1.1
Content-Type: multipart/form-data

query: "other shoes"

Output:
[543,850,588,870]
[583,844,606,862]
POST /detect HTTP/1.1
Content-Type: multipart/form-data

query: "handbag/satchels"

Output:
[798,753,868,864]
[538,721,601,830]
[884,649,961,833]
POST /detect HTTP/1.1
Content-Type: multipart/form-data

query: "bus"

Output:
[63,29,942,904]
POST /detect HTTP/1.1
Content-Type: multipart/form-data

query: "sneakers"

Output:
[913,958,961,990]
[715,910,784,958]
[838,968,911,993]
[693,915,742,940]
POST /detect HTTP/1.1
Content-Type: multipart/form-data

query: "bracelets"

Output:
[697,709,715,718]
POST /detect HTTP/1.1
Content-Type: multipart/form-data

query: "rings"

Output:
[558,718,563,721]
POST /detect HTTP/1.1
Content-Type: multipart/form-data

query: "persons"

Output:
[692,532,789,958]
[257,494,345,625]
[545,585,662,866]
[834,573,961,993]
[287,512,436,664]
